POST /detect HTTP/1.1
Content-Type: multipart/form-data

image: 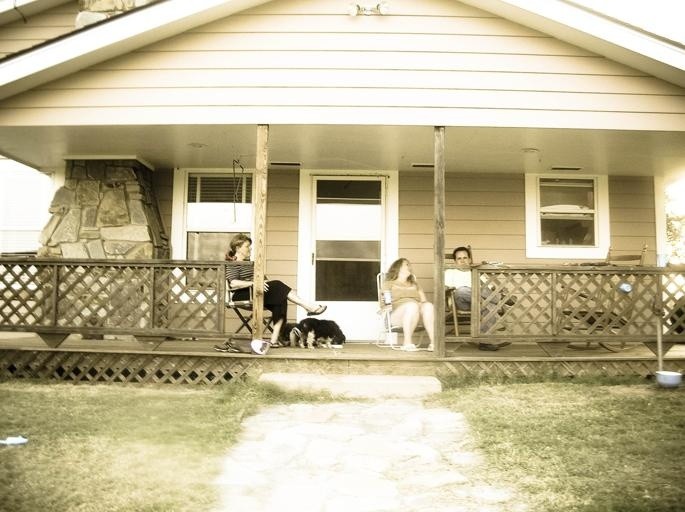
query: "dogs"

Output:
[292,317,346,349]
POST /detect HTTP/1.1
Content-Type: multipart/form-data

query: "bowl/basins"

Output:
[656,370,681,389]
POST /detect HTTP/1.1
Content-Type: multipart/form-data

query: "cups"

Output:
[382,290,392,302]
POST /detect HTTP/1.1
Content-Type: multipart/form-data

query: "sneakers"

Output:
[426,343,435,351]
[479,342,511,351]
[401,343,417,352]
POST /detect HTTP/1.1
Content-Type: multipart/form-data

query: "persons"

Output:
[378,259,436,351]
[225,234,327,348]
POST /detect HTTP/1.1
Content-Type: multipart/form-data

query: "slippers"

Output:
[269,341,279,348]
[307,305,327,316]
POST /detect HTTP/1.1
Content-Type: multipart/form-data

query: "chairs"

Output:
[222,265,289,348]
[373,271,438,352]
[551,241,651,352]
